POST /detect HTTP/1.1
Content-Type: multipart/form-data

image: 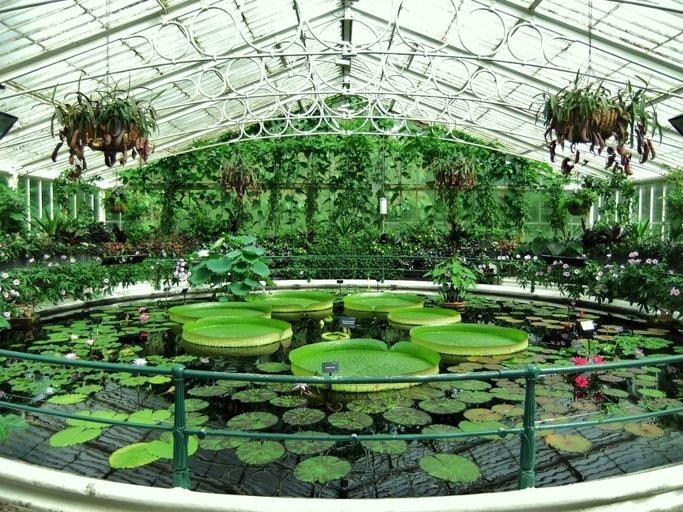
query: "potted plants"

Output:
[423,257,479,309]
[433,141,475,195]
[218,143,261,208]
[31,1,167,181]
[102,187,128,214]
[530,3,663,177]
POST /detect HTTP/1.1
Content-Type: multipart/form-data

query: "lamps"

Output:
[379,135,388,215]
